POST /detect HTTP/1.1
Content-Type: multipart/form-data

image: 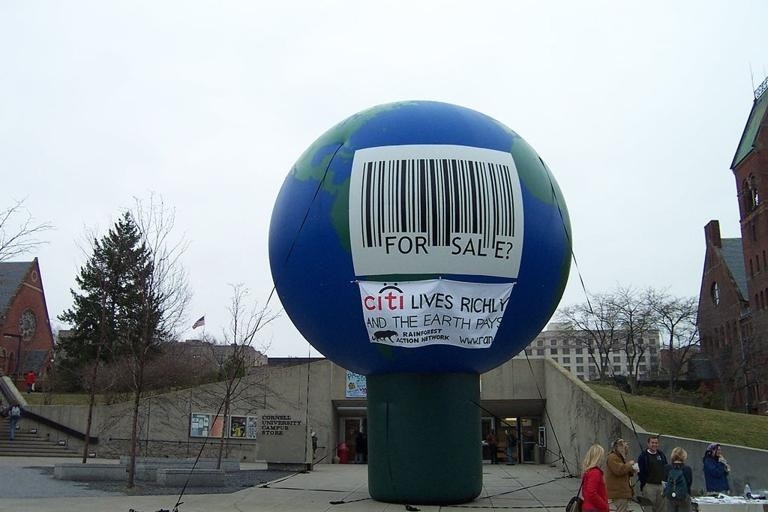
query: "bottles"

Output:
[744,483,752,502]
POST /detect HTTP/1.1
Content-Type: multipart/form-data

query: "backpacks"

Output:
[664,464,688,501]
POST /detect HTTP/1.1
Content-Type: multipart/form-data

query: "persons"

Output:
[702,443,732,495]
[605,437,639,511]
[25,370,37,394]
[346,428,367,463]
[8,400,21,439]
[580,442,609,511]
[505,428,519,465]
[663,446,693,511]
[637,435,668,511]
[484,428,501,465]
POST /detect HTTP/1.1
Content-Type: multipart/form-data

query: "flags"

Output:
[192,317,205,329]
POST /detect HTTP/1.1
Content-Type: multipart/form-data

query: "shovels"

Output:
[333,441,341,464]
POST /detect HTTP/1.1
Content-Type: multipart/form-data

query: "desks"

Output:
[691,493,768,512]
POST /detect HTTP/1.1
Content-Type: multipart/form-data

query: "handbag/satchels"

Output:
[566,497,583,512]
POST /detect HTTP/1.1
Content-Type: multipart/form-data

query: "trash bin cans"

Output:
[338,442,351,464]
[534,443,543,464]
